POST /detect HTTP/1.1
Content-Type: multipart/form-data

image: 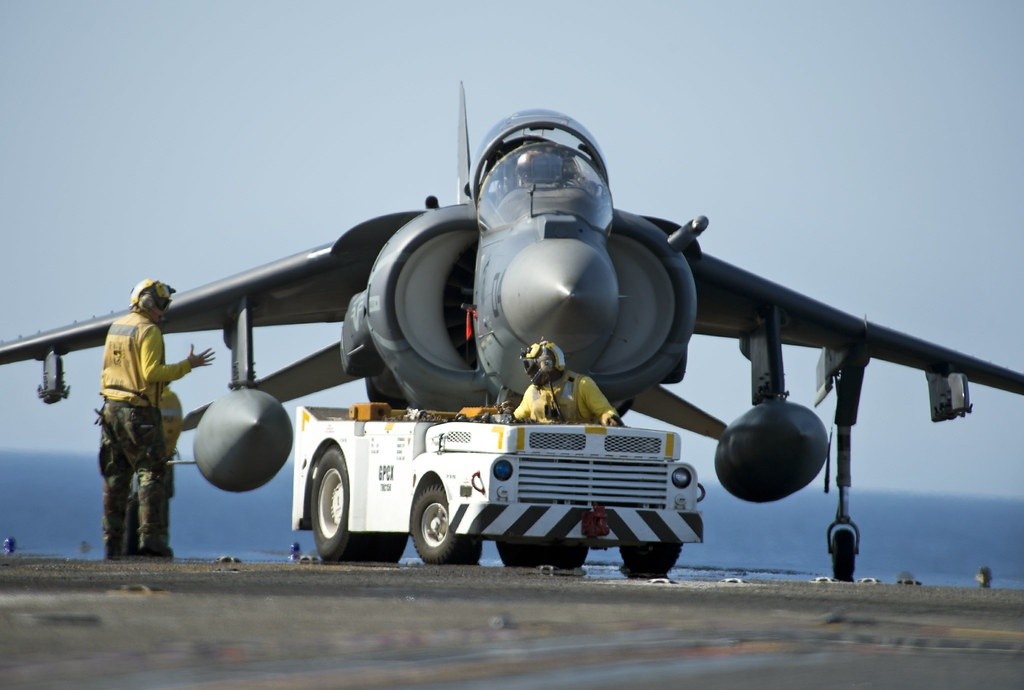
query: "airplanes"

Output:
[0,84,1024,583]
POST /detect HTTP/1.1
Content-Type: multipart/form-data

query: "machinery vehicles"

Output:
[289,402,707,577]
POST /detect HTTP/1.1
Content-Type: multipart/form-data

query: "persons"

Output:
[513,339,626,427]
[93,277,217,560]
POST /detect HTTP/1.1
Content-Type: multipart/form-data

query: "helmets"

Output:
[521,341,566,384]
[130,279,176,317]
